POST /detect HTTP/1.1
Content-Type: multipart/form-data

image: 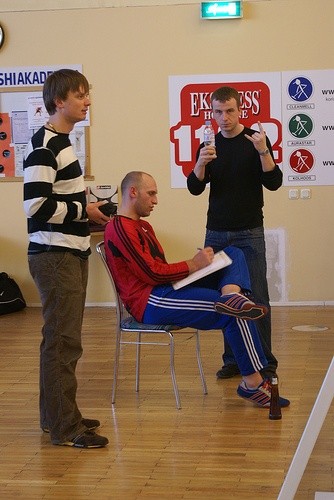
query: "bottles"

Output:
[269,375,282,420]
[204,121,215,157]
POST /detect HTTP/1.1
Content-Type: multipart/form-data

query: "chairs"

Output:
[96,240,208,409]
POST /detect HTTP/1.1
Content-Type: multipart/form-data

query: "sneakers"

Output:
[42,417,100,433]
[52,429,108,449]
[214,292,269,321]
[237,379,290,409]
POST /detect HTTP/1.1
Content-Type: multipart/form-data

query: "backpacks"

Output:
[0,272,26,315]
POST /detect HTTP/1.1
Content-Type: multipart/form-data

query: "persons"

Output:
[105,171,291,408]
[23,69,109,448]
[187,86,283,382]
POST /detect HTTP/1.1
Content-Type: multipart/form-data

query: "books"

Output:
[172,250,232,290]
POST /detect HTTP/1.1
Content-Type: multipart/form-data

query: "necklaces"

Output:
[47,121,56,131]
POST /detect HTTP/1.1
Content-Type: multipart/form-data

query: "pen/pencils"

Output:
[196,246,202,251]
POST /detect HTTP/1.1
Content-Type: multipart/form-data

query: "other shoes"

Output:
[216,364,240,378]
[268,373,278,385]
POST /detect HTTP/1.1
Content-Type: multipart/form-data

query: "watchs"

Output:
[259,148,269,155]
